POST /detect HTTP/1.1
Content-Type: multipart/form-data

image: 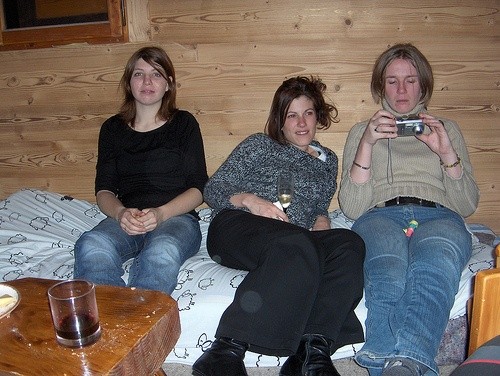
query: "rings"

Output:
[439,120,444,126]
[375,128,378,132]
[124,226,126,231]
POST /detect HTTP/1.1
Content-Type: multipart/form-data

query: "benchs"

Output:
[0,190,500,368]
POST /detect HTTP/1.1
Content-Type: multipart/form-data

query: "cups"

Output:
[48,278,101,348]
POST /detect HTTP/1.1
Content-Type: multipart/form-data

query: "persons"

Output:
[337,43,480,376]
[192,77,367,376]
[75,46,209,294]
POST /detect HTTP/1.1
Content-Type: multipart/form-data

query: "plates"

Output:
[0,283,21,318]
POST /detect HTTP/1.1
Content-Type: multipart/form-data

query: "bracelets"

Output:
[440,153,460,168]
[353,161,371,169]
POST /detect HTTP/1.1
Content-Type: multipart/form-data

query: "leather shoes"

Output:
[279,333,341,376]
[192,336,250,376]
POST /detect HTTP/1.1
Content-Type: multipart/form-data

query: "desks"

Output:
[0,277,181,376]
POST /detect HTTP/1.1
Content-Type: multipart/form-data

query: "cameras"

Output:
[391,113,425,137]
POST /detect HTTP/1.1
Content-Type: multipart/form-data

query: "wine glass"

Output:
[277,171,294,214]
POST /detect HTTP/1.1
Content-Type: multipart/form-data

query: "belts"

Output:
[374,196,443,207]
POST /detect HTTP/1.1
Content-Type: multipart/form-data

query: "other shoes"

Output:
[380,357,428,376]
